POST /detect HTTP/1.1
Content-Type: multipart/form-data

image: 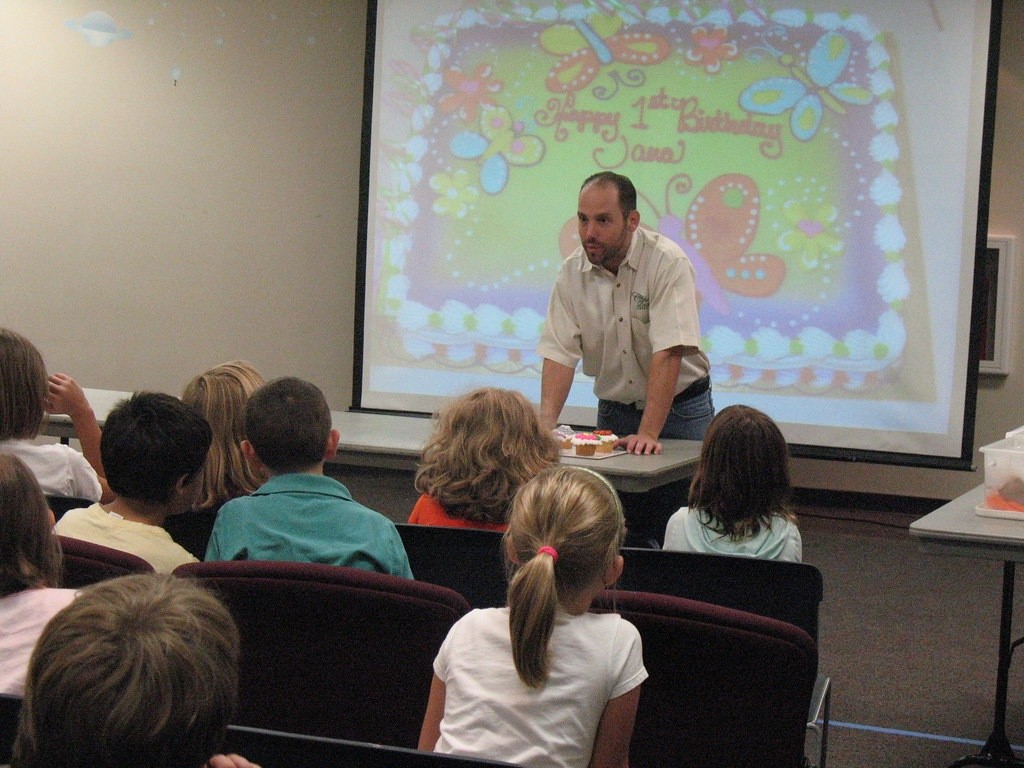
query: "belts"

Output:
[673,376,709,406]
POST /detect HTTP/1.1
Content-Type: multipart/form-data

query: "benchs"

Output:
[46,494,824,646]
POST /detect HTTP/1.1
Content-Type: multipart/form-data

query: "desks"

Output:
[41,387,704,547]
[909,484,1024,768]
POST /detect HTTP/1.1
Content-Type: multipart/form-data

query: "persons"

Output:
[177,359,266,517]
[0,454,90,700]
[415,463,650,768]
[51,391,212,578]
[0,325,116,504]
[538,172,717,536]
[661,405,803,564]
[409,387,559,534]
[12,572,264,768]
[204,377,417,581]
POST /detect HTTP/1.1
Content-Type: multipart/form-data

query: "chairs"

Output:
[0,536,833,768]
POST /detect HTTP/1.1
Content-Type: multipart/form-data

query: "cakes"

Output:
[376,0,909,390]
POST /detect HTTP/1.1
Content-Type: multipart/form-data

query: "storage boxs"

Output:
[974,429,1024,521]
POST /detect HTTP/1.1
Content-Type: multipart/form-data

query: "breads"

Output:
[997,474,1024,503]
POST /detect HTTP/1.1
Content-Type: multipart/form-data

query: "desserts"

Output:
[552,425,619,457]
